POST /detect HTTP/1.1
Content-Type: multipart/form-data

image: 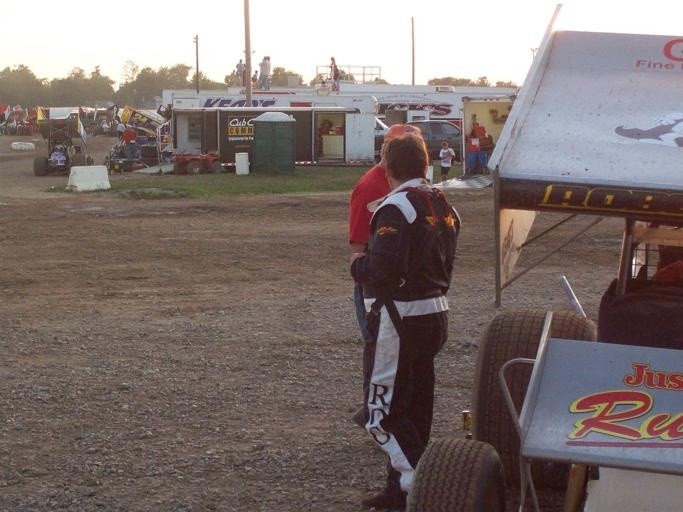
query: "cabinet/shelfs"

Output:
[321,134,344,158]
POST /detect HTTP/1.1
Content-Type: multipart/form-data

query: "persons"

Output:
[439,114,494,182]
[348,131,463,512]
[2,119,32,135]
[318,120,331,157]
[237,56,270,91]
[330,57,341,91]
[101,120,153,136]
[345,124,429,435]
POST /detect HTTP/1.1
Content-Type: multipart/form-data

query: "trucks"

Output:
[168,92,391,173]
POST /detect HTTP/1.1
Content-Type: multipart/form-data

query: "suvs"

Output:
[176,149,222,174]
[405,29,682,512]
[404,120,462,159]
[0,106,168,174]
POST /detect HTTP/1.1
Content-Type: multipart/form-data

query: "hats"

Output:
[383,124,421,143]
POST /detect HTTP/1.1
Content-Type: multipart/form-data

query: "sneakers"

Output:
[361,491,405,510]
